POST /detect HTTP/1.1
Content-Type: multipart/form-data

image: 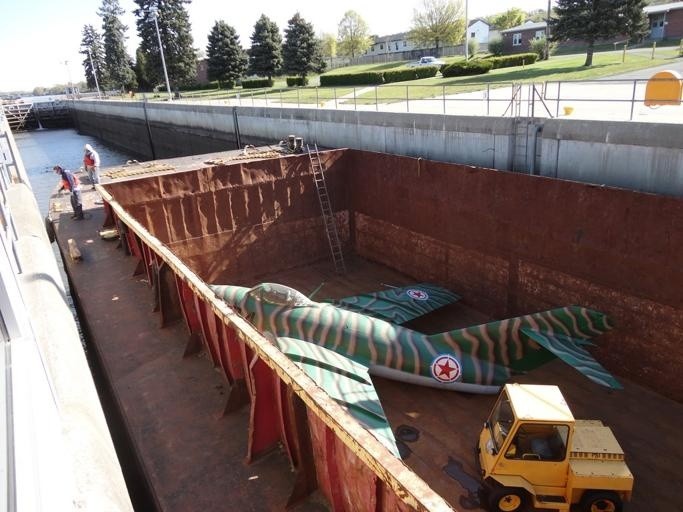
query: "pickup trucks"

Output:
[406,55,445,68]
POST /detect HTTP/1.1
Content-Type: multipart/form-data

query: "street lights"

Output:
[151,10,172,101]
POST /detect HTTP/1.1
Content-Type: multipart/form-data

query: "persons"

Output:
[82,144,100,189]
[52,165,84,220]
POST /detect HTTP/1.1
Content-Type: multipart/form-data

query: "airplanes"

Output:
[209,282,624,460]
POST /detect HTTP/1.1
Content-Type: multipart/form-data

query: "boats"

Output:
[46,135,683,512]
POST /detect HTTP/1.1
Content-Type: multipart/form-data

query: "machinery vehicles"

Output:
[476,384,634,512]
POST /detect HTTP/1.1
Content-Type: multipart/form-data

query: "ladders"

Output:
[307,142,347,276]
